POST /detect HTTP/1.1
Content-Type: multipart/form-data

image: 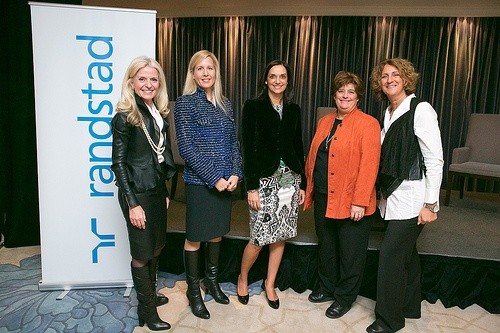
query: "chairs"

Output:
[167,101,249,200]
[317,106,362,126]
[444,113,500,206]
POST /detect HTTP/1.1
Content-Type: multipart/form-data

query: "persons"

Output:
[299,71,380,320]
[111,57,178,331]
[366,58,445,333]
[237,61,306,309]
[173,51,240,319]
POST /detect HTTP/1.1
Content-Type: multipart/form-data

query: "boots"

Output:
[201,240,229,305]
[149,256,169,307]
[130,261,172,331]
[183,247,211,319]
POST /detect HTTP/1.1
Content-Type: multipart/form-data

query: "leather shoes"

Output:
[325,298,357,319]
[367,319,393,333]
[307,288,335,303]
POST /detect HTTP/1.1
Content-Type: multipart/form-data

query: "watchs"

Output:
[423,203,438,213]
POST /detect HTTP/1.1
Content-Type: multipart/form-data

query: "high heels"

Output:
[237,274,250,305]
[263,277,280,310]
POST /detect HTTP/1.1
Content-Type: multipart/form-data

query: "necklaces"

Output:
[325,133,334,148]
[137,114,165,155]
